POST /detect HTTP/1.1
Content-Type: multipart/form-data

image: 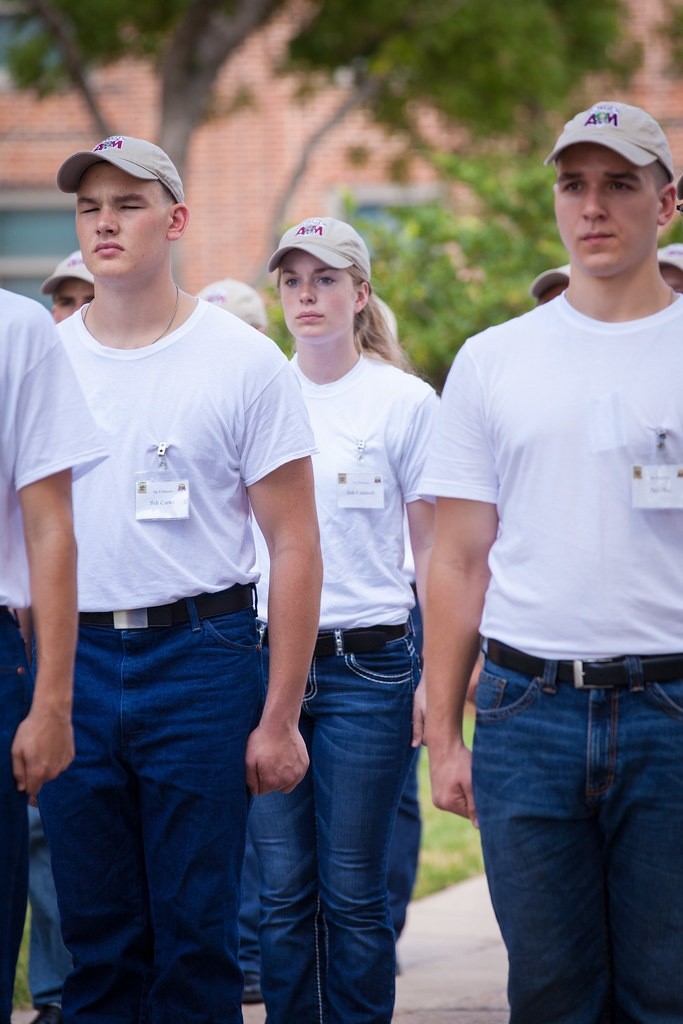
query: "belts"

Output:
[78,583,251,629]
[488,640,683,689]
[257,624,408,657]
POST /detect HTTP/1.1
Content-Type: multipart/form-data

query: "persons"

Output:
[196,278,270,338]
[0,286,105,1024]
[270,217,445,1023]
[426,101,683,1024]
[35,133,323,1024]
[40,247,94,325]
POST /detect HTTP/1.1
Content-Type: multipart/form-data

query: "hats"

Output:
[267,218,372,281]
[543,101,674,185]
[529,263,571,298]
[372,294,397,346]
[58,136,183,207]
[195,280,268,330]
[658,242,683,272]
[40,249,94,295]
